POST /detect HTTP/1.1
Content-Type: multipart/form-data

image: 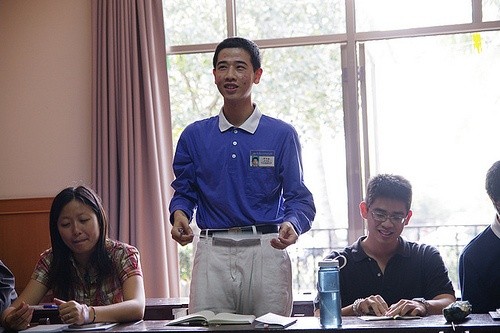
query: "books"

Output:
[166,310,256,326]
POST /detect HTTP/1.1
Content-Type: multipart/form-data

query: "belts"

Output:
[200,225,279,235]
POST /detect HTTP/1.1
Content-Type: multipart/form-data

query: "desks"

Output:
[0,290,500,333]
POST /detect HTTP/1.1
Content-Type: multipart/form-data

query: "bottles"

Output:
[317,254,347,327]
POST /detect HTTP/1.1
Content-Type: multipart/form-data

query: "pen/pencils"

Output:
[179,227,186,235]
[23,303,60,311]
[263,323,268,329]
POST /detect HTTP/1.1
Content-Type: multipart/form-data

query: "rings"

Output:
[66,313,71,319]
[16,314,19,318]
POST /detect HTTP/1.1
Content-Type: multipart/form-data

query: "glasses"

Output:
[369,205,408,224]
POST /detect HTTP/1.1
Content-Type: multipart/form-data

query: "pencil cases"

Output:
[442,300,473,322]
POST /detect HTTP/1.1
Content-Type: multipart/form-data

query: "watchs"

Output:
[412,297,431,316]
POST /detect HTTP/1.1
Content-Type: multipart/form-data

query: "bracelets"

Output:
[353,298,363,316]
[91,307,96,323]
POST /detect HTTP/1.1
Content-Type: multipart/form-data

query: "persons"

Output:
[0,260,18,315]
[312,173,456,317]
[169,36,316,317]
[459,160,500,313]
[0,185,145,331]
[252,157,259,166]
